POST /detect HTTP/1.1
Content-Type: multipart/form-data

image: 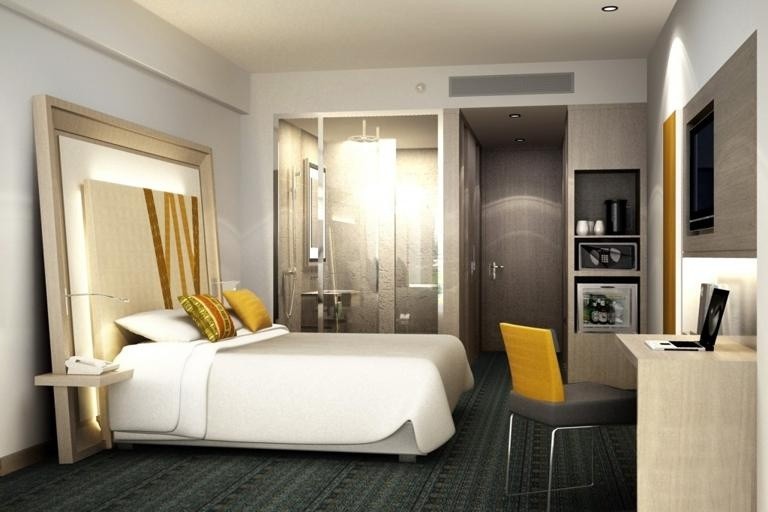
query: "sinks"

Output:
[301,290,361,322]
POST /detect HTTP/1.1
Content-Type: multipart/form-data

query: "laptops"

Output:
[644,287,730,352]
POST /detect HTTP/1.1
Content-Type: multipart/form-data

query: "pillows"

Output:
[115,306,245,342]
[177,294,237,342]
[222,289,271,332]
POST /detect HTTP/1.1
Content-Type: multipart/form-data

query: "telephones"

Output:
[64,355,119,375]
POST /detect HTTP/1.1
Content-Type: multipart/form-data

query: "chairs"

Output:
[499,322,635,512]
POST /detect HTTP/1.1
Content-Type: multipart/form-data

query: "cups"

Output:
[577,220,606,236]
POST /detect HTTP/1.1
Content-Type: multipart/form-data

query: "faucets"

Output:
[311,274,331,279]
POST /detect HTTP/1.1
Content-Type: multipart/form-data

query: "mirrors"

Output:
[304,158,327,268]
[83,179,474,463]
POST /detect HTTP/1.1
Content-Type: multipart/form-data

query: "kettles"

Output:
[602,198,627,235]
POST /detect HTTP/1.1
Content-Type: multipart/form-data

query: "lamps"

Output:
[66,293,129,302]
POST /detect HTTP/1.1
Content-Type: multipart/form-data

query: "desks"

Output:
[34,369,133,386]
[611,332,758,512]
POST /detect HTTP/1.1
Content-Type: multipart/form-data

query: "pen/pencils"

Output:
[664,348,699,351]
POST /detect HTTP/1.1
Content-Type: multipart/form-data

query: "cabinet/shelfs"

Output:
[574,168,640,335]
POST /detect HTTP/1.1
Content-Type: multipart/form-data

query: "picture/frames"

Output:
[578,242,638,271]
[576,283,639,333]
